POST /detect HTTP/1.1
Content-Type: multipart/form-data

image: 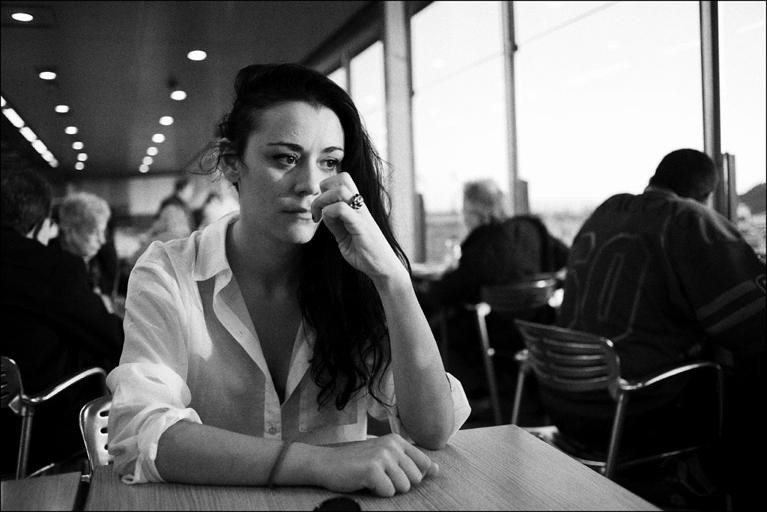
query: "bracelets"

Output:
[264,434,295,491]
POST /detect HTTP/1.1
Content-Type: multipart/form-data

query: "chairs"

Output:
[438,272,557,426]
[512,318,732,511]
[0,354,110,481]
[78,394,113,485]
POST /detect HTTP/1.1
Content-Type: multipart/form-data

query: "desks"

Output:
[0,423,665,511]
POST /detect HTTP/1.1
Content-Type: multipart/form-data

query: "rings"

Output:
[350,191,364,210]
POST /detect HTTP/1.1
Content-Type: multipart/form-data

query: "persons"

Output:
[43,191,117,313]
[0,166,125,483]
[553,148,767,512]
[419,176,569,426]
[130,176,226,266]
[102,60,476,496]
